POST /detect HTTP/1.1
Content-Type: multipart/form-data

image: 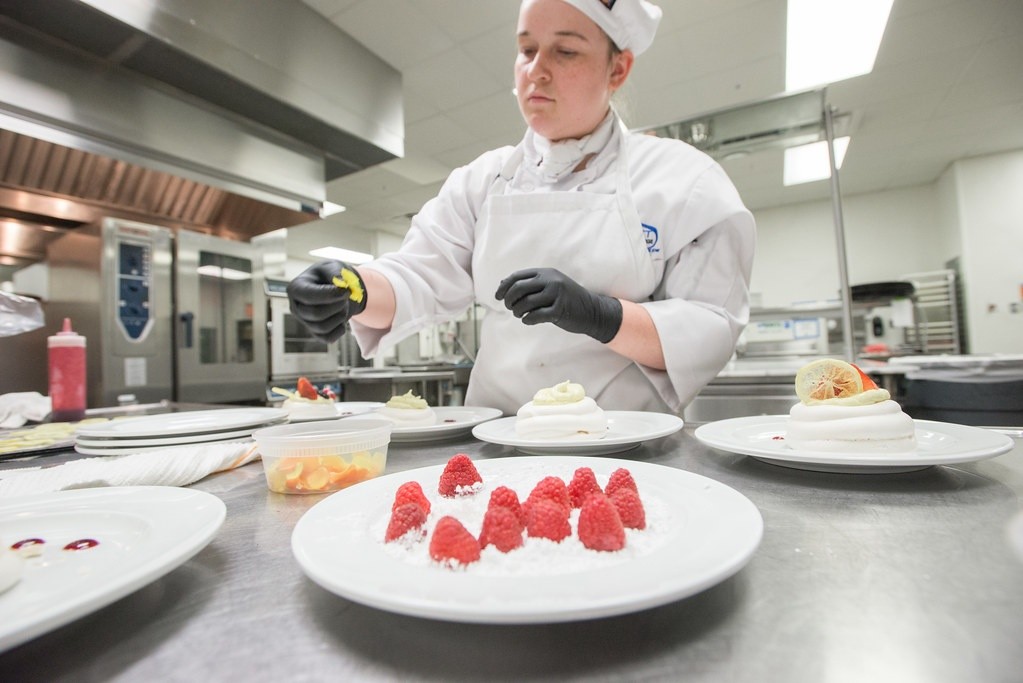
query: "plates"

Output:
[472,410,684,456]
[73,409,291,457]
[291,457,764,625]
[695,415,1015,474]
[343,407,503,442]
[284,401,385,421]
[2,486,227,654]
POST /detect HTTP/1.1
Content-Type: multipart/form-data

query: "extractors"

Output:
[3,1,407,248]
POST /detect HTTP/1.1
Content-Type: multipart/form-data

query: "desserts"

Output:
[784,358,914,441]
[271,377,341,418]
[514,380,608,441]
[379,389,435,427]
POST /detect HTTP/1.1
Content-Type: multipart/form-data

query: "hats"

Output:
[560,0,662,59]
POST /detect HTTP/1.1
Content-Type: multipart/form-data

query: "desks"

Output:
[686,358,920,402]
[0,403,1023,682]
[340,371,454,406]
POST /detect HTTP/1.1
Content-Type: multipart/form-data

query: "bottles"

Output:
[47,317,86,422]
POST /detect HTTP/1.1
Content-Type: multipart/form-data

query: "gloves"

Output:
[494,267,624,344]
[286,259,368,344]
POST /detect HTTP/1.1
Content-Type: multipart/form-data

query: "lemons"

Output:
[0,417,132,452]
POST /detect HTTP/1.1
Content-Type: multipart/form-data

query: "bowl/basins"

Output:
[253,420,392,494]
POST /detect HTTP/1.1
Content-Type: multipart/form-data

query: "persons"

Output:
[286,1,757,417]
